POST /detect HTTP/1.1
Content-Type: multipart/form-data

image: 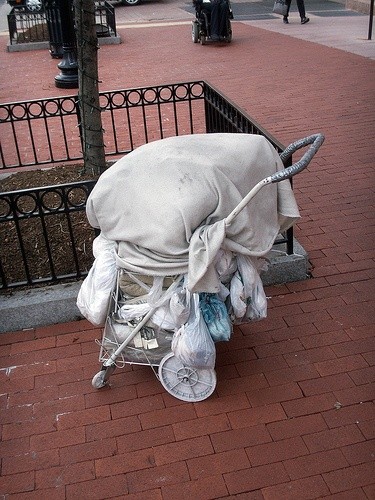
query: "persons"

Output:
[193,0,230,41]
[283,0,311,24]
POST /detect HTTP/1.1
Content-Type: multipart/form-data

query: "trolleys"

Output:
[89,134,326,402]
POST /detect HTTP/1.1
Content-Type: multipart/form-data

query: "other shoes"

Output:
[300,17,310,24]
[282,18,290,24]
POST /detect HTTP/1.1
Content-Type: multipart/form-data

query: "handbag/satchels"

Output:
[272,0,288,16]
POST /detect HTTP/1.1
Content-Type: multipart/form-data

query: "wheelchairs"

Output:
[191,0,233,46]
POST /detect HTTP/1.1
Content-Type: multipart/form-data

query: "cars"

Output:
[5,0,162,14]
